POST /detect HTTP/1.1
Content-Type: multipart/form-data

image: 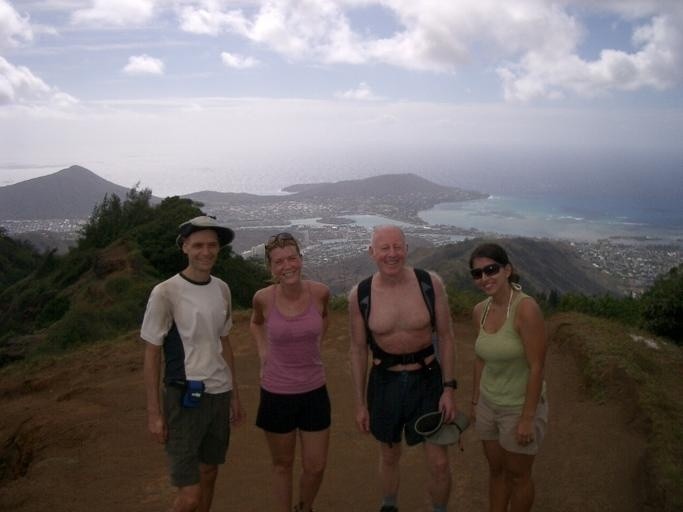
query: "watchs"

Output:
[443,379,458,390]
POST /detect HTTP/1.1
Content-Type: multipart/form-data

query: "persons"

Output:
[347,225,458,512]
[140,215,247,512]
[250,234,332,512]
[470,242,549,512]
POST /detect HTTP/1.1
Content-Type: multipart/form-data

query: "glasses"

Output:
[265,233,293,249]
[471,264,505,279]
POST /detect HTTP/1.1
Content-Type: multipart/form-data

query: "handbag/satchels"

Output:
[183,382,204,409]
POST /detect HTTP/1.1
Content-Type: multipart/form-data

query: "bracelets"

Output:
[471,398,479,405]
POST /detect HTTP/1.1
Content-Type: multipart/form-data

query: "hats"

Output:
[415,408,470,445]
[176,216,234,248]
